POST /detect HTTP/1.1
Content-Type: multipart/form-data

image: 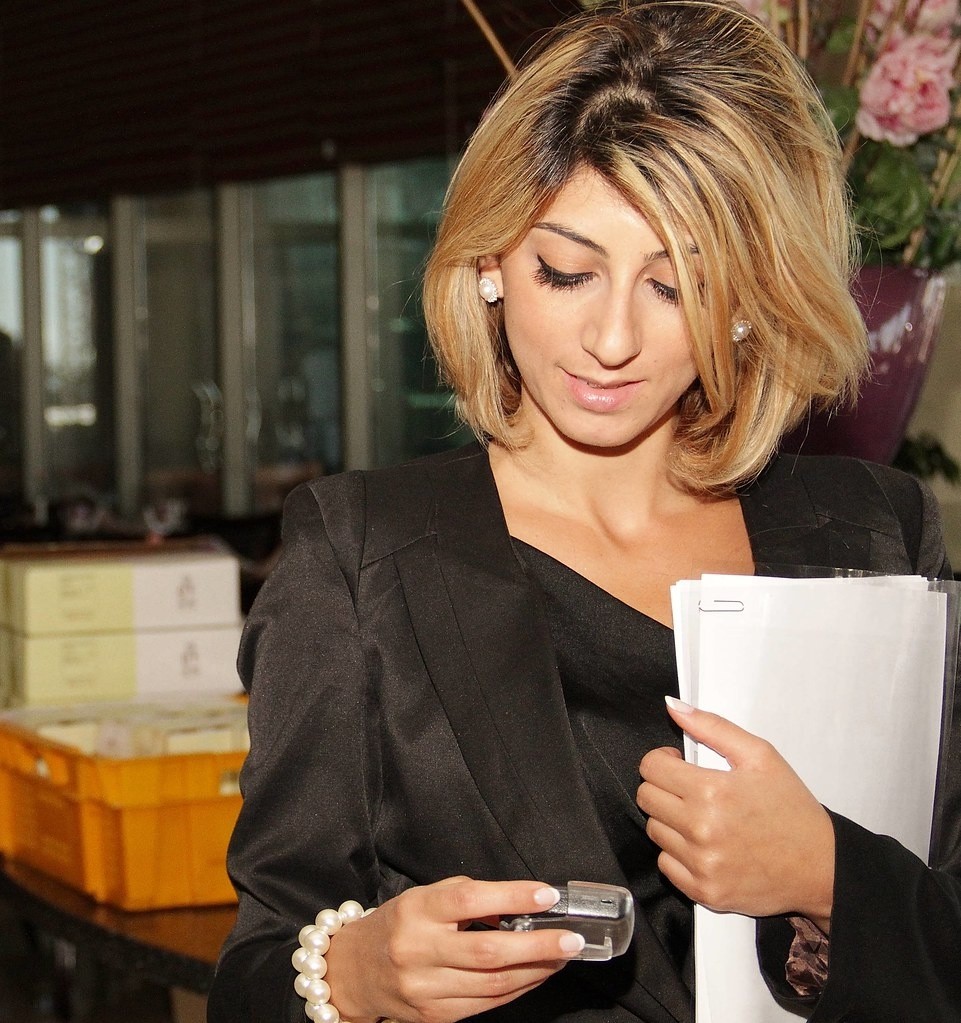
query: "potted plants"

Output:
[462,0,960,473]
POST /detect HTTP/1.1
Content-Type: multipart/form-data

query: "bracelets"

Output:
[293,899,394,1023]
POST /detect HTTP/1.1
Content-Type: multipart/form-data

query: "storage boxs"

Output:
[1,702,261,911]
[2,536,249,702]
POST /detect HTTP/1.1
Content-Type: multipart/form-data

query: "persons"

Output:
[203,2,961,1021]
